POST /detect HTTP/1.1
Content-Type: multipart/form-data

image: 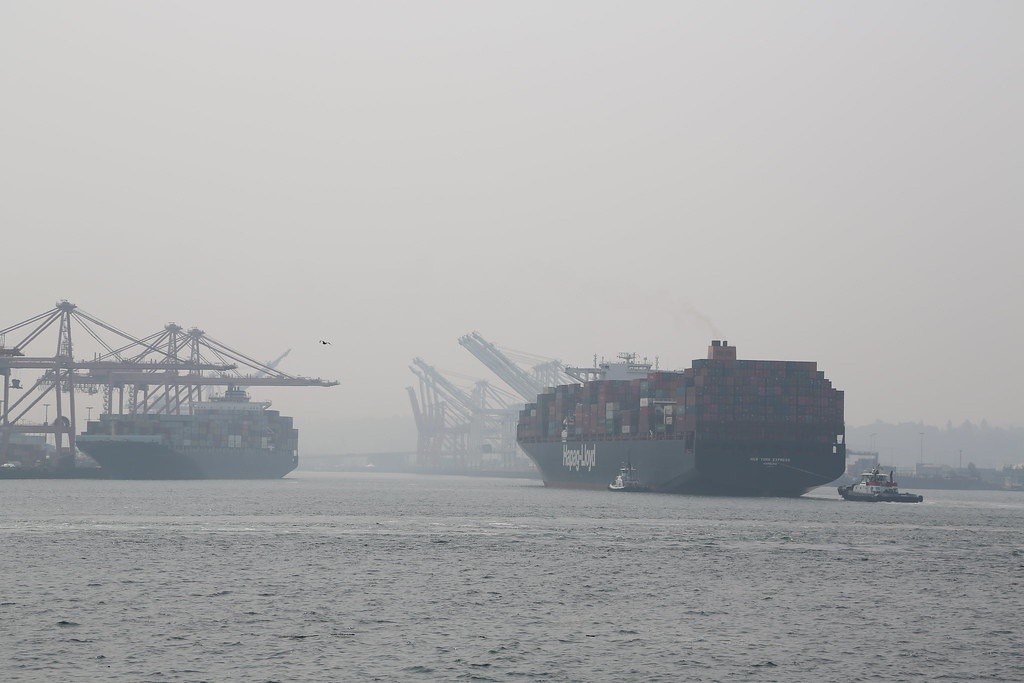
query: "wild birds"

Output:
[319,339,332,345]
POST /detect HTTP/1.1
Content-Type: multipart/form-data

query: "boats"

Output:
[838,461,924,503]
[76,382,300,481]
[608,455,648,493]
[515,341,846,499]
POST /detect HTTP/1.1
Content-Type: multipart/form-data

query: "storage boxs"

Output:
[82,410,298,452]
[519,359,844,446]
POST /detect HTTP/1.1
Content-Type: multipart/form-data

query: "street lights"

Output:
[87,406,94,420]
[43,403,52,422]
[920,431,924,464]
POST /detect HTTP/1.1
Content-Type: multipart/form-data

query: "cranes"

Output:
[0,298,344,465]
[406,329,593,475]
